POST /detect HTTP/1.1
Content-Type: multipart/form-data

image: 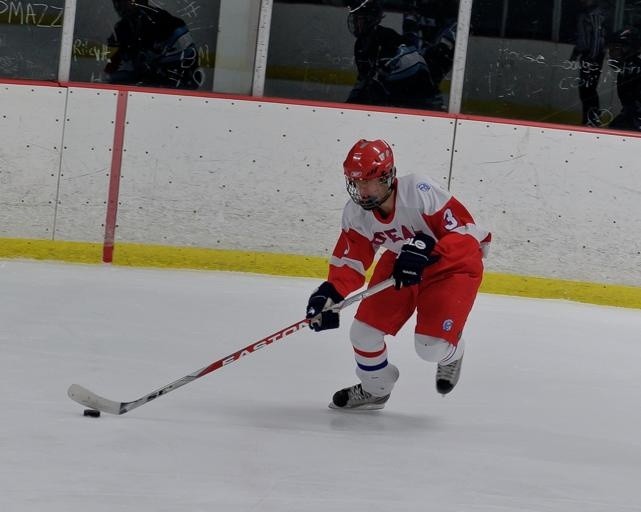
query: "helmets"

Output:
[347,0,384,25]
[609,26,641,69]
[344,139,396,189]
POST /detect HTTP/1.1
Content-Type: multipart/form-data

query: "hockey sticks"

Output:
[67,277,395,414]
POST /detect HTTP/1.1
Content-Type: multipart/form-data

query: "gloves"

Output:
[304,282,344,332]
[391,233,436,290]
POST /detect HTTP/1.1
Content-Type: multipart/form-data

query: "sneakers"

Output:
[436,355,464,394]
[333,382,392,408]
[584,112,602,128]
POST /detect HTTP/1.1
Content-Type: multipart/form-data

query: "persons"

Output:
[569,0,610,128]
[400,0,459,86]
[604,25,641,133]
[304,137,493,410]
[100,0,201,90]
[341,0,445,112]
